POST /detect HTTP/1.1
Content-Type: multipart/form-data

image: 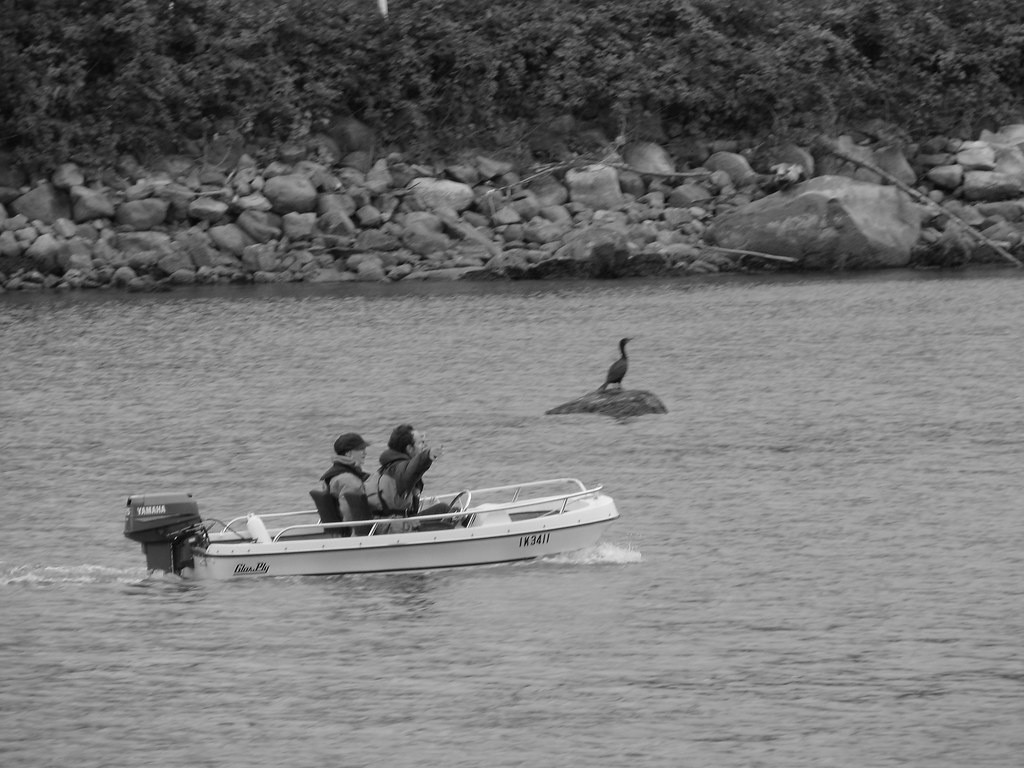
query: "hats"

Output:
[334,432,371,455]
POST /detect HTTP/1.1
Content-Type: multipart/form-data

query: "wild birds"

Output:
[595,337,635,394]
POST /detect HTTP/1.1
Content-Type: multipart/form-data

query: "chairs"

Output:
[343,492,378,536]
[309,491,352,534]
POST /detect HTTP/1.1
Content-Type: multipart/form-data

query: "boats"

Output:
[120,477,620,583]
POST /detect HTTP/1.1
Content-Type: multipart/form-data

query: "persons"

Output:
[373,425,461,535]
[320,433,371,536]
